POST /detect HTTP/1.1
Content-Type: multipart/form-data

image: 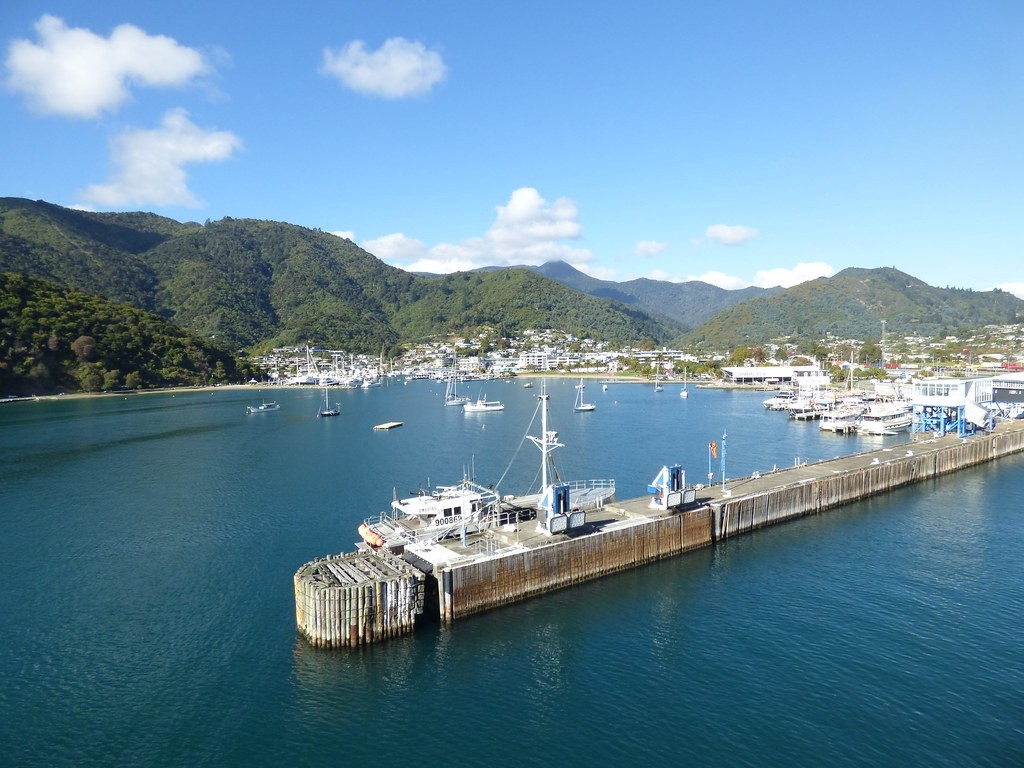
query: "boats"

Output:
[763,366,913,435]
[355,378,616,545]
[463,390,504,413]
[245,398,280,417]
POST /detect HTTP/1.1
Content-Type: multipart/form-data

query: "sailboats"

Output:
[315,388,341,417]
[267,344,381,387]
[654,364,664,392]
[679,366,689,400]
[443,350,471,406]
[573,377,596,412]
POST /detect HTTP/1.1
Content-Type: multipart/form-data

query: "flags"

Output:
[710,442,718,460]
